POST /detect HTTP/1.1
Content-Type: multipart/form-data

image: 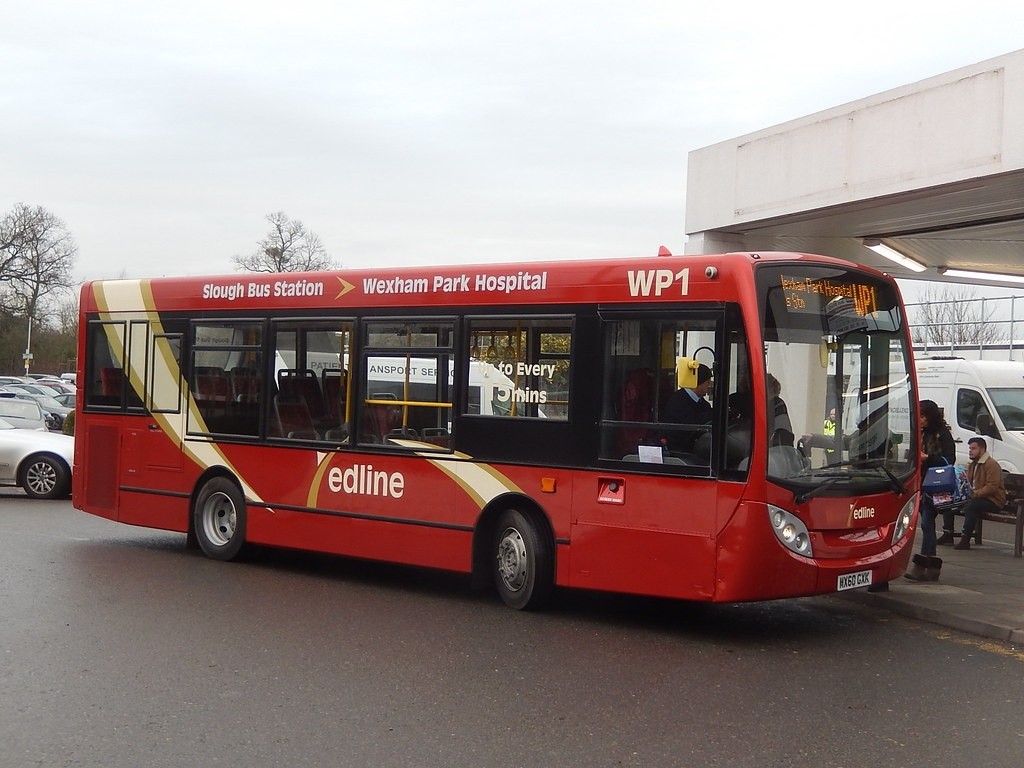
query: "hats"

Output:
[698,364,712,384]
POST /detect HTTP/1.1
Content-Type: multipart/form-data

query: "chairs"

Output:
[615,368,674,458]
[99,365,452,452]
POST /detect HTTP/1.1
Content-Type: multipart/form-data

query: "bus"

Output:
[68,242,921,613]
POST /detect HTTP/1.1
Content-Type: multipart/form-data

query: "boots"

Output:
[919,557,942,581]
[904,554,926,580]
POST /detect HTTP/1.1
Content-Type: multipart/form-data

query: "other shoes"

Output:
[954,537,971,549]
[936,531,954,546]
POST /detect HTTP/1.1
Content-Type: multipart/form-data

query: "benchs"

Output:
[938,498,1024,557]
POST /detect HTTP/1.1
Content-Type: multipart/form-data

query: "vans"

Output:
[843,360,1024,514]
[275,348,548,418]
[61,373,76,385]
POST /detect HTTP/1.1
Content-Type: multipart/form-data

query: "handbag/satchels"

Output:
[924,455,973,511]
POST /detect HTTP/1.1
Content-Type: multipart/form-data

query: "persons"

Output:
[803,407,895,464]
[935,438,1006,550]
[904,400,956,579]
[660,363,713,452]
[824,408,843,463]
[766,373,793,446]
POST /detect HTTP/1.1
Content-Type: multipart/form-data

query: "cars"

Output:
[53,393,76,409]
[31,394,75,430]
[36,378,73,382]
[0,386,31,398]
[35,382,76,393]
[0,376,36,386]
[16,395,54,431]
[5,384,60,397]
[0,398,49,432]
[24,374,60,379]
[0,419,75,500]
